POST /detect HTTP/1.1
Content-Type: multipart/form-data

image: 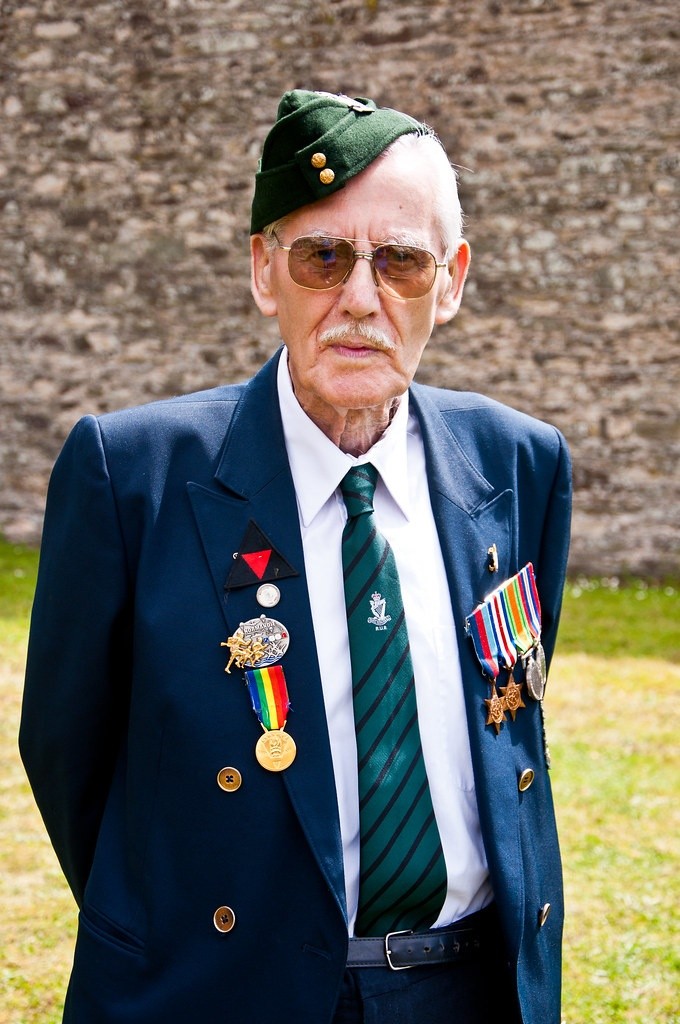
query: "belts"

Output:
[344,921,508,971]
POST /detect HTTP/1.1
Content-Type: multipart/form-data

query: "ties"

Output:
[337,462,447,940]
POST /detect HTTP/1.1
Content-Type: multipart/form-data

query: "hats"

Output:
[250,87,422,236]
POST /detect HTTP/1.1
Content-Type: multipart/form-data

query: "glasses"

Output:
[269,225,449,298]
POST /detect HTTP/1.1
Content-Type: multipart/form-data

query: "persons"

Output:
[18,91,573,1024]
